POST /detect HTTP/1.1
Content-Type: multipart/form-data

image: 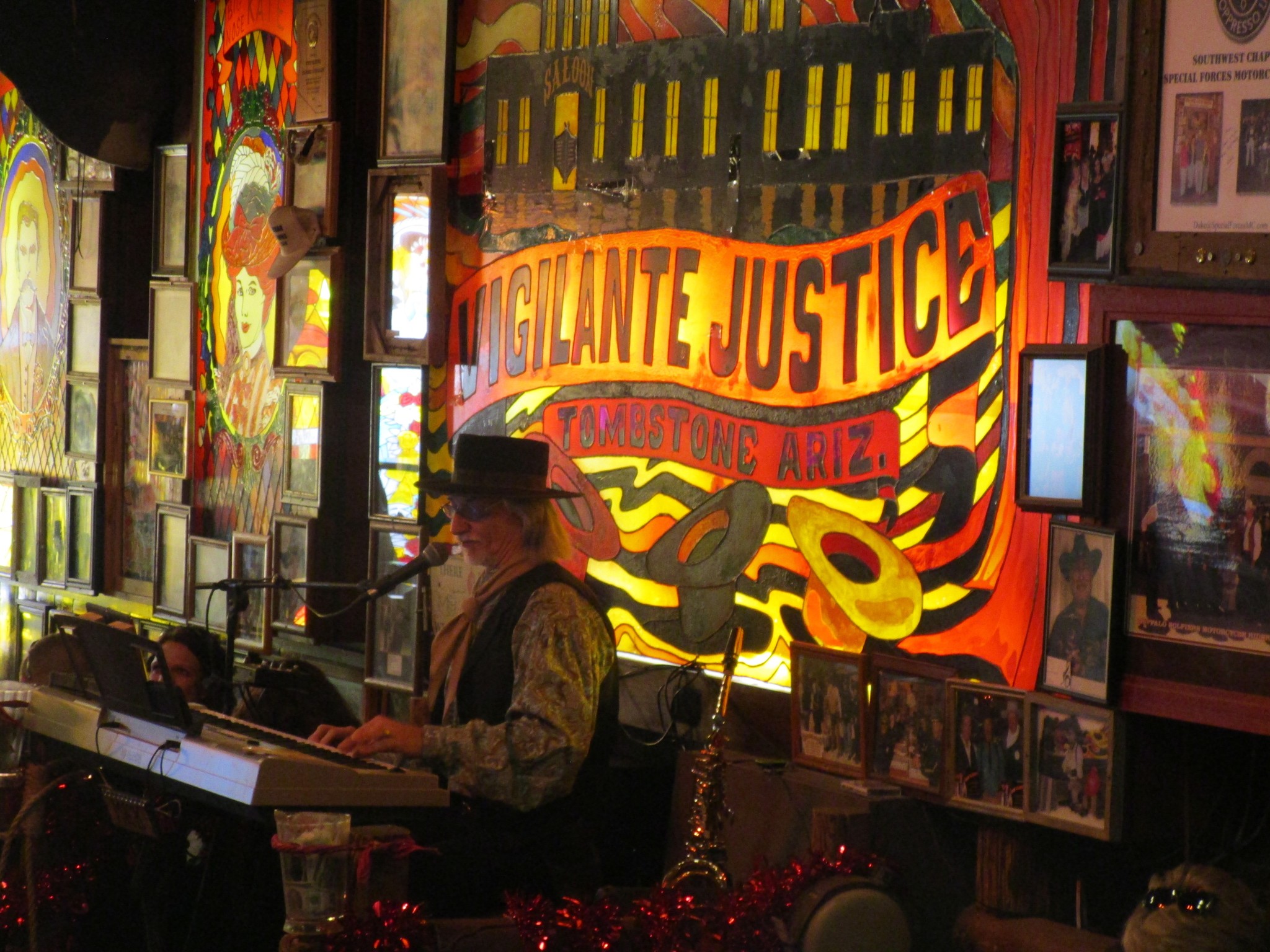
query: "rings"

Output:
[383,726,395,739]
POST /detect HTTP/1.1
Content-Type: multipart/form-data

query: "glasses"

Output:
[439,496,502,522]
[1143,889,1213,918]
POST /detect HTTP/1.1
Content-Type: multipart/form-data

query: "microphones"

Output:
[357,542,449,602]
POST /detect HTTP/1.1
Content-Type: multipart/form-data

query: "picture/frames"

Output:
[1042,520,1119,703]
[944,679,1029,820]
[1015,342,1109,513]
[870,648,953,795]
[1026,691,1117,840]
[789,640,866,776]
[1048,100,1120,284]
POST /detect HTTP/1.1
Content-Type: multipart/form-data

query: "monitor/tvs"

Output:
[1014,342,1102,508]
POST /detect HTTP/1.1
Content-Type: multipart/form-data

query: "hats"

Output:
[412,434,585,503]
[1059,534,1102,580]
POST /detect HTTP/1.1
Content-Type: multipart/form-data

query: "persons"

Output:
[1142,454,1263,627]
[874,679,944,790]
[1120,862,1270,952]
[1059,127,1121,274]
[274,528,306,626]
[1046,531,1107,684]
[146,625,235,714]
[308,433,620,918]
[372,536,416,680]
[232,659,361,739]
[800,668,862,769]
[1037,716,1100,819]
[955,700,1023,811]
